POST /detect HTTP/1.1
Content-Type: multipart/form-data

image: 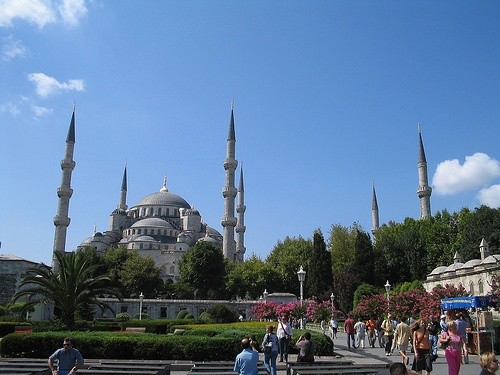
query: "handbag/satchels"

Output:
[286,334,291,340]
[297,355,307,362]
[261,345,272,353]
[440,332,449,349]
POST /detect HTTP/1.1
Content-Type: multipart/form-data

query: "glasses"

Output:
[282,319,285,320]
[490,360,498,363]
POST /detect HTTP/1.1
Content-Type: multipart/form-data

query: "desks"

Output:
[466,332,492,355]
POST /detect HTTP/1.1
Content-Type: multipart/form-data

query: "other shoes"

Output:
[405,357,409,365]
[347,348,350,351]
[352,345,357,349]
[386,352,392,356]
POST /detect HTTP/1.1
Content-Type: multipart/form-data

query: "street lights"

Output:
[384,280,391,319]
[297,265,307,330]
[330,292,335,316]
[139,292,144,320]
[263,288,269,321]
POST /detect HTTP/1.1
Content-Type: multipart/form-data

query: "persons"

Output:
[296,332,314,362]
[411,317,432,375]
[261,325,278,375]
[376,316,385,347]
[366,316,376,349]
[462,310,472,328]
[478,351,497,375]
[277,316,292,363]
[390,362,419,375]
[328,316,338,339]
[440,311,465,375]
[321,319,327,336]
[381,313,394,356]
[234,338,259,375]
[429,315,442,361]
[344,315,356,351]
[48,337,84,375]
[394,316,410,365]
[353,318,366,349]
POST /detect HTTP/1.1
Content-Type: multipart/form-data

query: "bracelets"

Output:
[73,366,77,371]
